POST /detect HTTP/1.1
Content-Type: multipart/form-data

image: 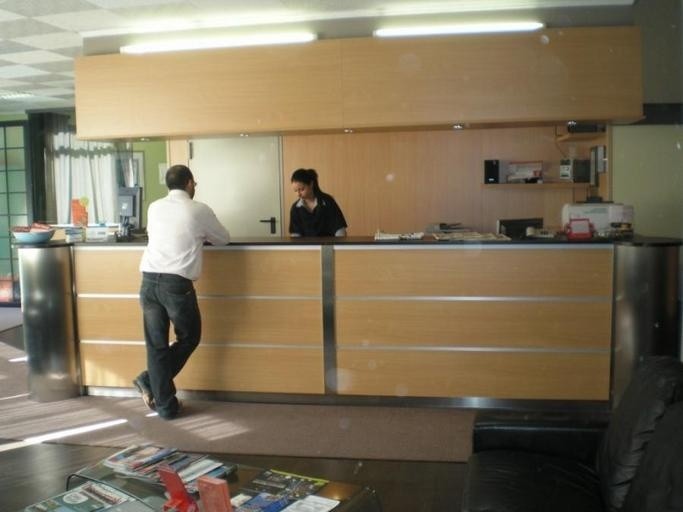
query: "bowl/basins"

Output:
[11,226,56,244]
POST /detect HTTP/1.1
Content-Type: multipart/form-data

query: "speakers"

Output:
[560,159,590,182]
[485,159,499,183]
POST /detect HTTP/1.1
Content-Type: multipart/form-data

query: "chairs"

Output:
[464,355,681,511]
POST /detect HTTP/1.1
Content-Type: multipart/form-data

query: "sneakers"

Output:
[175,399,184,414]
[132,377,154,411]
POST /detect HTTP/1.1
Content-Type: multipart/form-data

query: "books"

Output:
[373,233,425,241]
[32,444,341,512]
[433,229,511,242]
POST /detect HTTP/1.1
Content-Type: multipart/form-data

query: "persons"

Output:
[289,168,349,238]
[132,165,229,419]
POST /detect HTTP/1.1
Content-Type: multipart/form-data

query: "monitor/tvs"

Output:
[117,187,142,243]
[496,217,543,237]
